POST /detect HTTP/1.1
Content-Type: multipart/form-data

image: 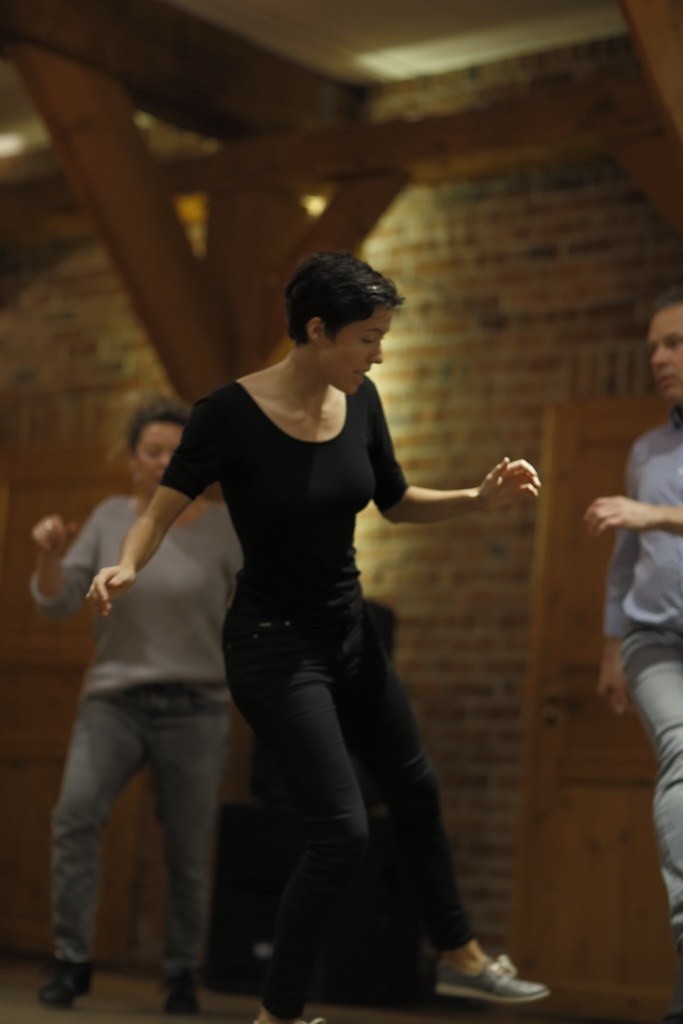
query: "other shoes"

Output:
[164,982,199,1014]
[38,981,75,1006]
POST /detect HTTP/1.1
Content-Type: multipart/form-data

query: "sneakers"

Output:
[251,1016,327,1024]
[435,953,550,1006]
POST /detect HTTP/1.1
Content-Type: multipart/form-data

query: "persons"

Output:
[83,251,549,1024]
[29,402,243,1014]
[582,285,682,1024]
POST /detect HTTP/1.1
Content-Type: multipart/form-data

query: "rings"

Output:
[44,519,53,528]
